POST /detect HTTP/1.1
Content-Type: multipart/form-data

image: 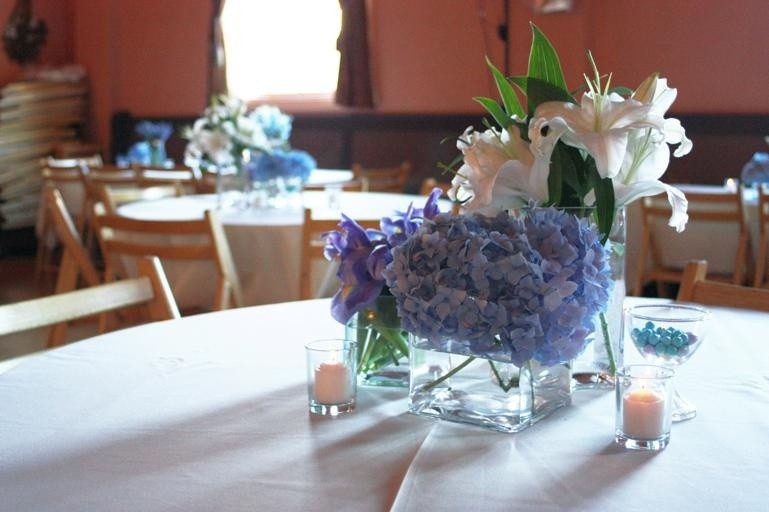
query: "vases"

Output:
[212,172,268,212]
[339,207,630,434]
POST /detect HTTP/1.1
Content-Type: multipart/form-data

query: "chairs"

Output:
[353,160,412,192]
[1,151,242,338]
[301,207,379,300]
[631,181,769,312]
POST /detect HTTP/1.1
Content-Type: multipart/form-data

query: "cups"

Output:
[613,363,672,452]
[304,338,360,417]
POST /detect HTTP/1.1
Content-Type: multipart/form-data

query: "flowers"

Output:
[324,21,691,368]
[186,94,315,193]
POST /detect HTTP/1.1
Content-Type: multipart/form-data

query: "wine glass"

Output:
[626,303,711,424]
